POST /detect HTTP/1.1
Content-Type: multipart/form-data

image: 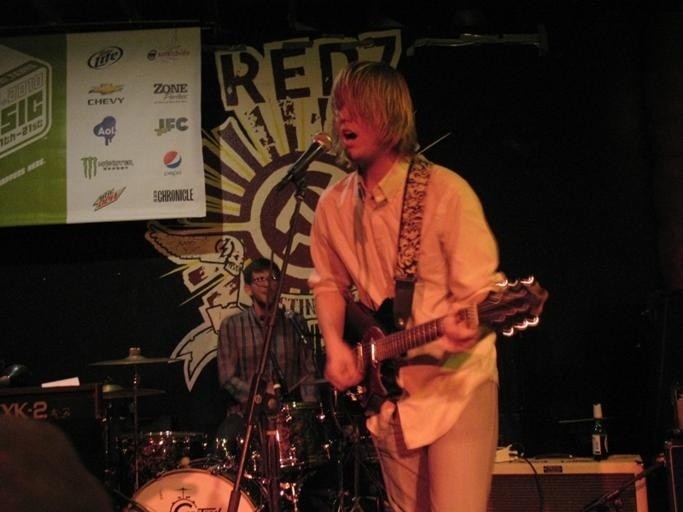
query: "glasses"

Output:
[248,273,280,286]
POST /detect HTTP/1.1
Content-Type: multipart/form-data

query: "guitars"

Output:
[314,279,548,416]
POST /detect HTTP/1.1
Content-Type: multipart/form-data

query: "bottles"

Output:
[589,400,610,460]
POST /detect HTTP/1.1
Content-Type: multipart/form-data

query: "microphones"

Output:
[275,130,336,185]
[283,308,310,346]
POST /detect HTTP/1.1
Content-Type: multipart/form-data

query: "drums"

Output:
[260,401,334,468]
[109,433,204,476]
[120,464,268,512]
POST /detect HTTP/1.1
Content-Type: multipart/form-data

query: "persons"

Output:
[306,60,509,512]
[215,258,317,461]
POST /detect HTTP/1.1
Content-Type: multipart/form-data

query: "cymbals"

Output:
[91,357,174,365]
[100,385,165,398]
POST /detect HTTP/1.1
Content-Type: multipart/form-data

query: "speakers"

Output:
[485,454,650,511]
